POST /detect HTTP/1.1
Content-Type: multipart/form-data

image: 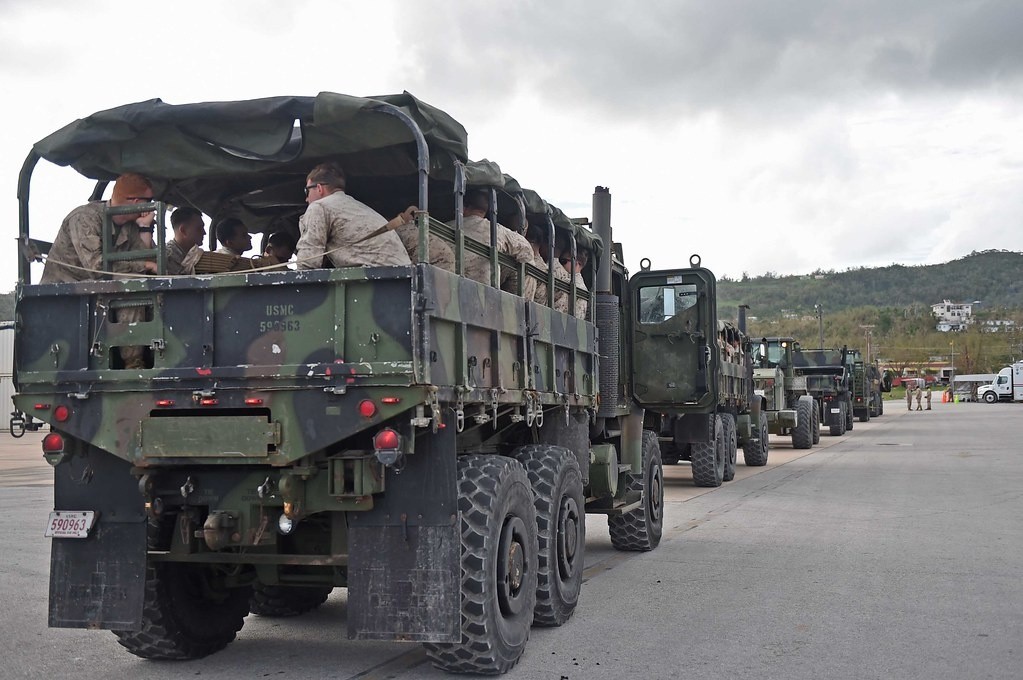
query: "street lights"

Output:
[948,340,955,404]
[859,324,876,364]
[813,302,824,349]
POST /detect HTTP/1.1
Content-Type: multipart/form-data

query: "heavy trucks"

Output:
[8,85,727,678]
[846,347,886,422]
[750,335,822,450]
[794,348,854,437]
[638,286,770,488]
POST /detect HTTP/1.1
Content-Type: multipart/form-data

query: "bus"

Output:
[873,362,956,390]
[952,374,998,402]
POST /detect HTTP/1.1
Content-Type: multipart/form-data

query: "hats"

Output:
[926,385,930,387]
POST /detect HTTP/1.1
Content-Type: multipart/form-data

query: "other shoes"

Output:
[925,408,931,410]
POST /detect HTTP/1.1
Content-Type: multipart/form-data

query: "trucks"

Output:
[977,360,1022,404]
[900,379,925,390]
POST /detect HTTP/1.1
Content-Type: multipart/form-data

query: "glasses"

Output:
[126,197,153,203]
[559,258,571,265]
[304,182,330,197]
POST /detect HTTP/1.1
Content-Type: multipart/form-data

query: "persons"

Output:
[397,220,455,271]
[296,162,411,269]
[906,385,913,411]
[38,174,157,369]
[443,191,534,286]
[719,332,744,363]
[166,207,207,274]
[213,218,296,273]
[925,385,931,410]
[973,383,979,402]
[915,386,922,410]
[945,384,953,402]
[499,215,588,320]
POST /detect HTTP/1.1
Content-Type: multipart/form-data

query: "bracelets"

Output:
[139,227,153,232]
